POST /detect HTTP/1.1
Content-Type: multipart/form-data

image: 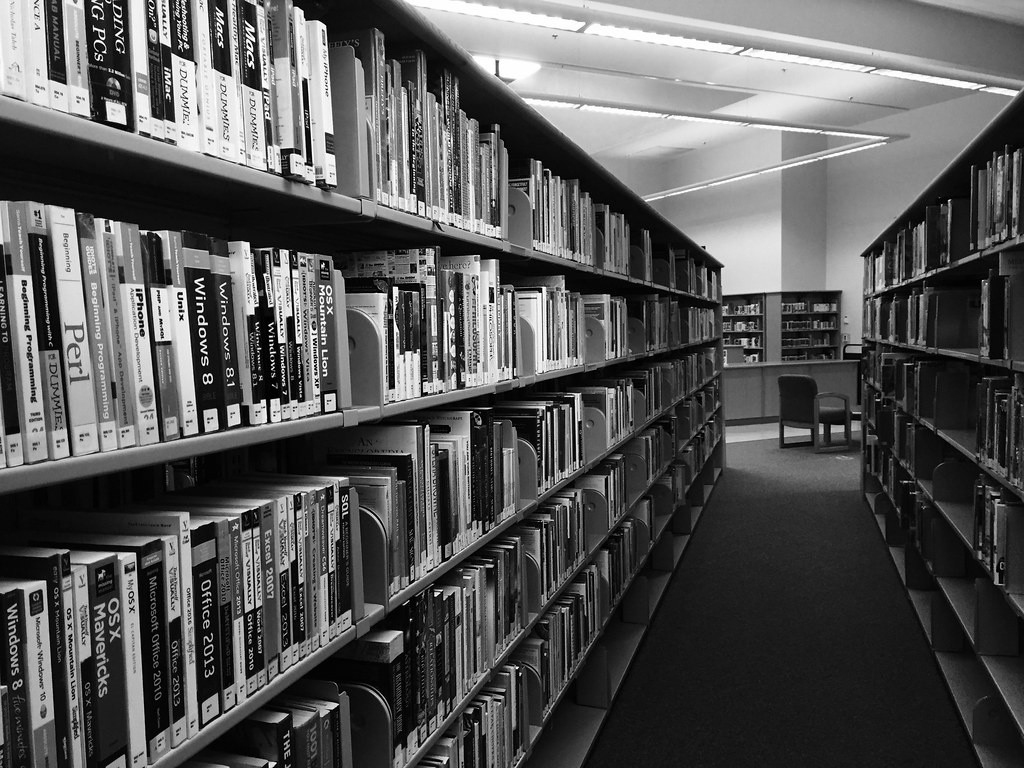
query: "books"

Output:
[722,301,839,365]
[863,145,1024,587]
[0,0,719,768]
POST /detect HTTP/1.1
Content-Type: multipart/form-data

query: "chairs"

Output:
[778,374,853,454]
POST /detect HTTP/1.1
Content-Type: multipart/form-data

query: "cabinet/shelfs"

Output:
[0,0,727,768]
[722,292,765,365]
[766,290,842,362]
[859,85,1024,768]
